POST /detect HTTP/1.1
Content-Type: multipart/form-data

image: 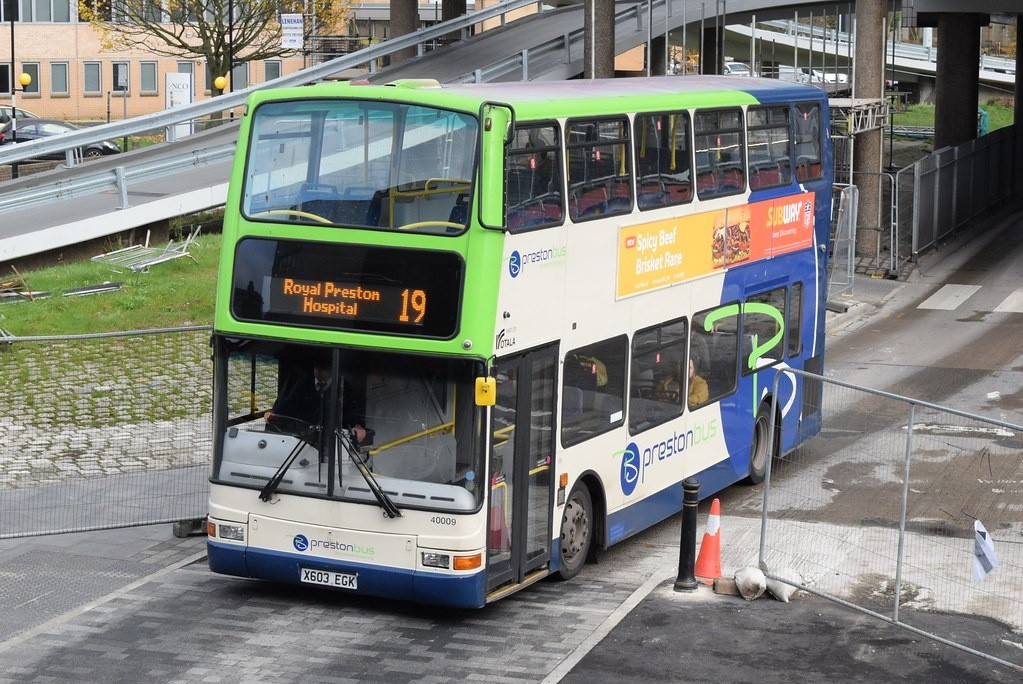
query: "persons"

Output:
[670,323,710,378]
[652,359,709,408]
[264,356,366,444]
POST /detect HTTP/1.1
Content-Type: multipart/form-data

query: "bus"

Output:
[202,77,835,617]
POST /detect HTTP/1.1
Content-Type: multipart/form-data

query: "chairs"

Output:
[290,139,820,233]
[563,320,780,442]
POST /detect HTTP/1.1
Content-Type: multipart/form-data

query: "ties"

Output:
[318,383,327,395]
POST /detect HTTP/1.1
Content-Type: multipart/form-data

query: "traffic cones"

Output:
[691,498,740,591]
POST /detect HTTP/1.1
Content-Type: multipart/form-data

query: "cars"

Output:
[0,119,122,162]
[0,106,42,134]
[726,63,757,78]
[798,68,848,86]
